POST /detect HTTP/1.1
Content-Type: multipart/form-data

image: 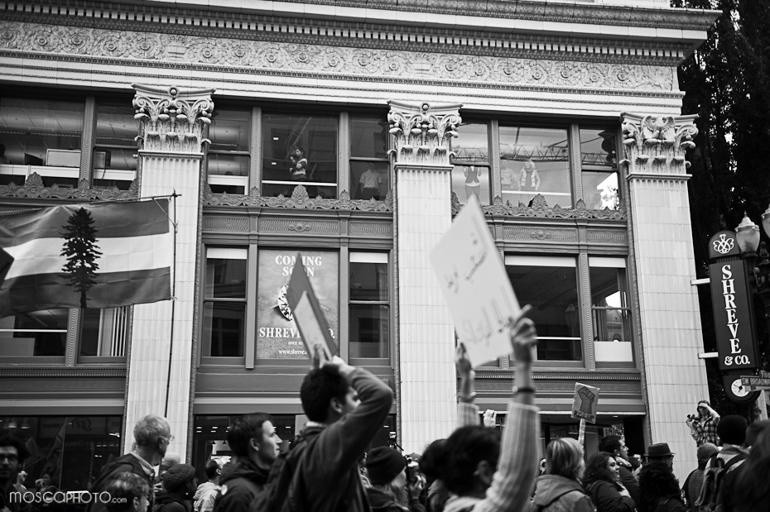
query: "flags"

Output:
[0,196,172,319]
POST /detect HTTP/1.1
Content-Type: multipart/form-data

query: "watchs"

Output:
[511,385,536,394]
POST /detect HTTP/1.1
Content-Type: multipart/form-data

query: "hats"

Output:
[697,443,719,462]
[644,442,675,457]
[717,415,746,445]
[365,445,406,486]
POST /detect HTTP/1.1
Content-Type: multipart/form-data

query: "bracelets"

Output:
[456,391,478,403]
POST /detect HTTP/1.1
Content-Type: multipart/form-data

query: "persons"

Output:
[441,303,542,512]
[517,161,541,191]
[463,153,482,203]
[1,414,770,511]
[685,399,721,447]
[359,162,383,201]
[251,354,395,511]
[288,145,309,182]
[499,161,519,192]
